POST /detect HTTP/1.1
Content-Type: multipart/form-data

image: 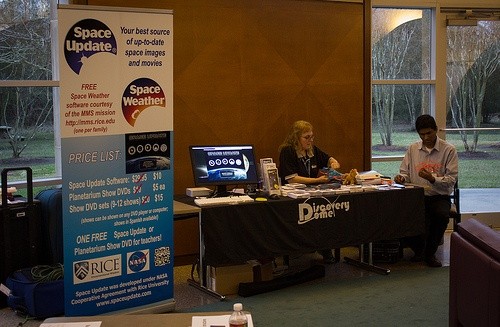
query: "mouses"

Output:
[255,197,267,202]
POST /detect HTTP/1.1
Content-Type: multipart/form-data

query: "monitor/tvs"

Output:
[190,143,260,198]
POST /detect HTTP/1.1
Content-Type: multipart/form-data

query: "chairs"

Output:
[449,218,500,327]
[449,178,461,232]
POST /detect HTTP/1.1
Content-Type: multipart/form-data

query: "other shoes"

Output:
[391,249,416,262]
[432,256,442,267]
[323,252,335,266]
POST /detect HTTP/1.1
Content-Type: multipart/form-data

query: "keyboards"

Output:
[193,195,254,207]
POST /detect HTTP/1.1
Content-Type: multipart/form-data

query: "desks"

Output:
[173,181,427,302]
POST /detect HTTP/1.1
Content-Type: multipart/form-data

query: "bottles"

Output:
[380,176,391,186]
[229,302,248,327]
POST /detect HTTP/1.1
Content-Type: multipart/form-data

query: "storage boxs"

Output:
[246,260,272,282]
[206,265,253,295]
[359,240,404,263]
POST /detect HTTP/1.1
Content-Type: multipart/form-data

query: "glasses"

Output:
[300,136,315,140]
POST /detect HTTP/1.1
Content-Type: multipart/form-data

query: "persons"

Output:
[278,120,340,264]
[395,115,459,266]
